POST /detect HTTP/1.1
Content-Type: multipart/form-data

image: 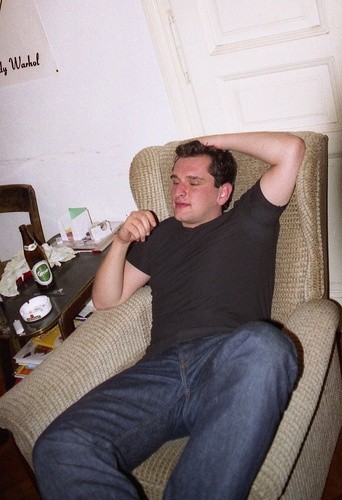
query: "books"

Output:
[13,319,85,378]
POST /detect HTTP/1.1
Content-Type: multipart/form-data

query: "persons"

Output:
[32,131,306,500]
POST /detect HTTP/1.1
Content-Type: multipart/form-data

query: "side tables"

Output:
[0,233,114,390]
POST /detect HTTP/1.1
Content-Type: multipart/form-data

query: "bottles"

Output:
[0,295,9,328]
[19,225,57,290]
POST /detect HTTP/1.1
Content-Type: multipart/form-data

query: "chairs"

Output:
[0,184,46,284]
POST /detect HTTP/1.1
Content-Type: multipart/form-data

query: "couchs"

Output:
[0,131,342,500]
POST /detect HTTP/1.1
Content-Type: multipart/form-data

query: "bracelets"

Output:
[115,227,129,244]
